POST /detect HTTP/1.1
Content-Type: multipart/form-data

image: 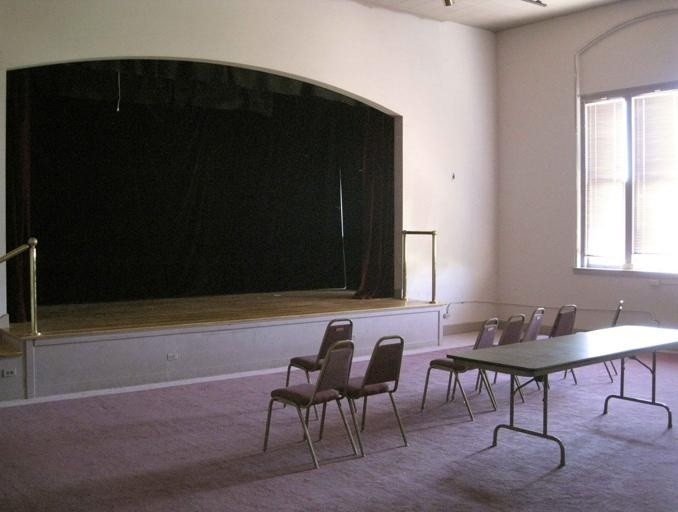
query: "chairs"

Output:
[419,299,625,420]
[258,316,414,471]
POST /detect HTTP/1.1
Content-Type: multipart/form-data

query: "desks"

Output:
[446,324,678,472]
